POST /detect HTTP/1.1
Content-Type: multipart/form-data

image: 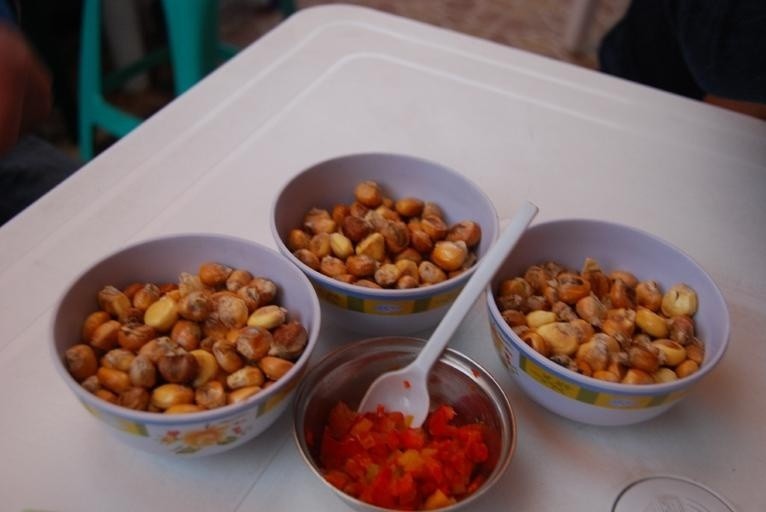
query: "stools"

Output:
[77,1,296,165]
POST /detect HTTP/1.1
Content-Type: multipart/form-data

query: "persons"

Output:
[0,1,81,224]
[594,0,766,126]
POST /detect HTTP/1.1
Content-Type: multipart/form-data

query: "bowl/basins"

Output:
[46,228,325,460]
[286,333,517,511]
[482,215,732,429]
[270,149,502,342]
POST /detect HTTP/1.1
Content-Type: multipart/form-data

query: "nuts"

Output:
[63,179,704,415]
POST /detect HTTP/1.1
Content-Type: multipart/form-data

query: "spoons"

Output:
[352,200,539,429]
